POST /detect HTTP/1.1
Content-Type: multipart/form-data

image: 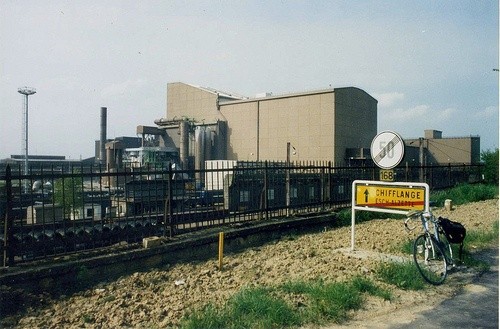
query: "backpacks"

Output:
[436,216,466,246]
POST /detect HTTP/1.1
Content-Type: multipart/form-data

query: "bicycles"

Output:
[404,206,463,286]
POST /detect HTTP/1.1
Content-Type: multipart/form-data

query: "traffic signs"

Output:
[352,181,428,215]
